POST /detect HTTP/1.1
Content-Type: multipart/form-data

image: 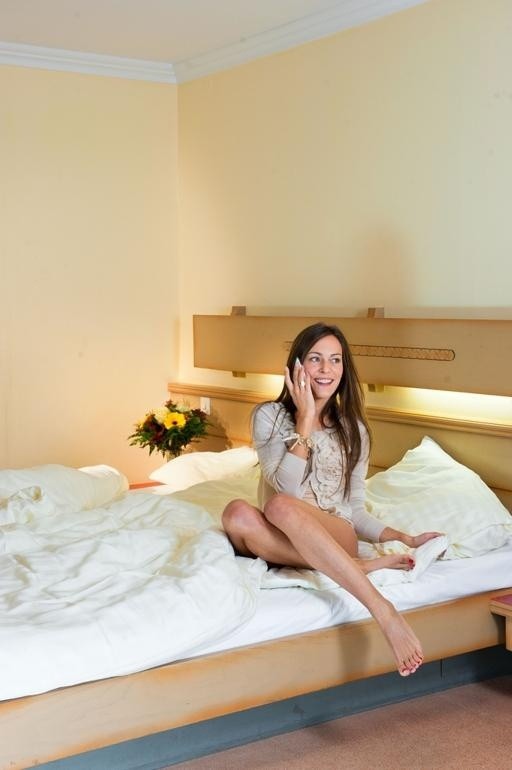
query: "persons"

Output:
[218,320,449,682]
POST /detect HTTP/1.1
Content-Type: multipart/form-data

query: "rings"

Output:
[299,382,305,386]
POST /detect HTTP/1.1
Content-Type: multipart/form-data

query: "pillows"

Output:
[152,443,260,491]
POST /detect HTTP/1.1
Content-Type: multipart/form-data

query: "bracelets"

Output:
[288,429,313,449]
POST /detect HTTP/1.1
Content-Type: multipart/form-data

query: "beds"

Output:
[0,461,512,769]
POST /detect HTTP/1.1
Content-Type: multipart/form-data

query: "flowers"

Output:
[126,398,215,462]
